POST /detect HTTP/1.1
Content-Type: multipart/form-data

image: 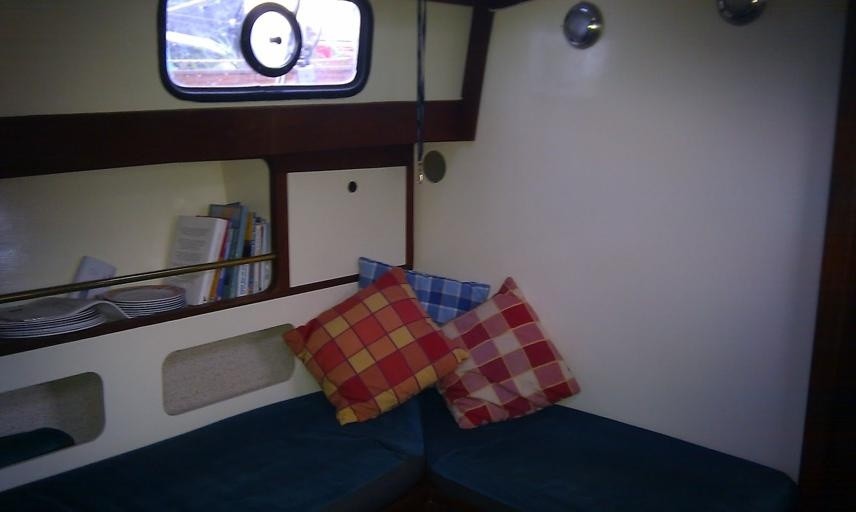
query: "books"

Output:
[164,202,270,306]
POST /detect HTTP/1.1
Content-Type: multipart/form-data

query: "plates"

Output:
[101,285,188,315]
[0,305,106,340]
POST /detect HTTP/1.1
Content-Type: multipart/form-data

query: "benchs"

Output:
[428,404,798,510]
[0,392,425,512]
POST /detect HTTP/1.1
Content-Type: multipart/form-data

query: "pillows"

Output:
[436,276,580,431]
[357,256,491,324]
[282,266,466,425]
